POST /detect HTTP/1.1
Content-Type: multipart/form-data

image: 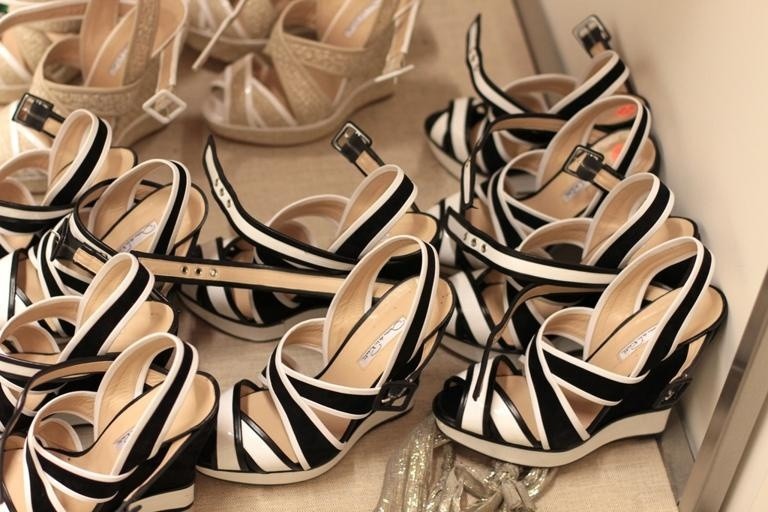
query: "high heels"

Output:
[422,12,639,179]
[438,172,703,368]
[2,0,455,511]
[429,236,727,467]
[428,96,662,276]
